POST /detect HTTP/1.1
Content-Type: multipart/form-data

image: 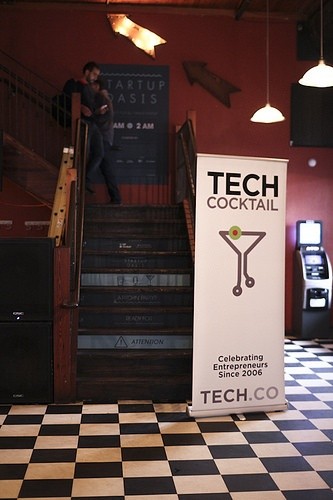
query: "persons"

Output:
[62,61,122,208]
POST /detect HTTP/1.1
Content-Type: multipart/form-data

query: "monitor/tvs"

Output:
[303,254,324,266]
[297,220,322,246]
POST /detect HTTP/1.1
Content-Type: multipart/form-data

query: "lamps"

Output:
[296,0,333,88]
[248,0,285,124]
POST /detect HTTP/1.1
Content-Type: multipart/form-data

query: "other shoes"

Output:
[110,198,121,204]
[85,184,97,196]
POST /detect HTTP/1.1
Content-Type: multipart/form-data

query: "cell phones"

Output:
[101,105,109,110]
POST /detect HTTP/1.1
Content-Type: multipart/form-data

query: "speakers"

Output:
[1,321,54,406]
[1,236,56,322]
